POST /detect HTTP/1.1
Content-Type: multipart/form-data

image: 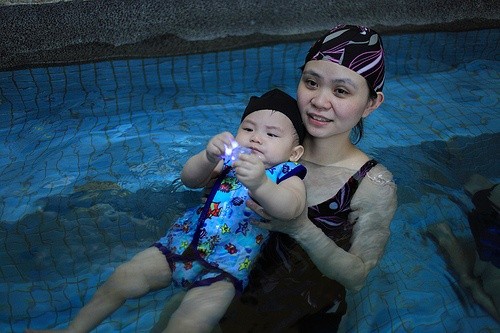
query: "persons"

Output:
[23,88,308,333]
[421,173,500,324]
[148,22,398,333]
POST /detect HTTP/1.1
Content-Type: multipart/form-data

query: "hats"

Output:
[304,25,385,94]
[241,89,306,146]
